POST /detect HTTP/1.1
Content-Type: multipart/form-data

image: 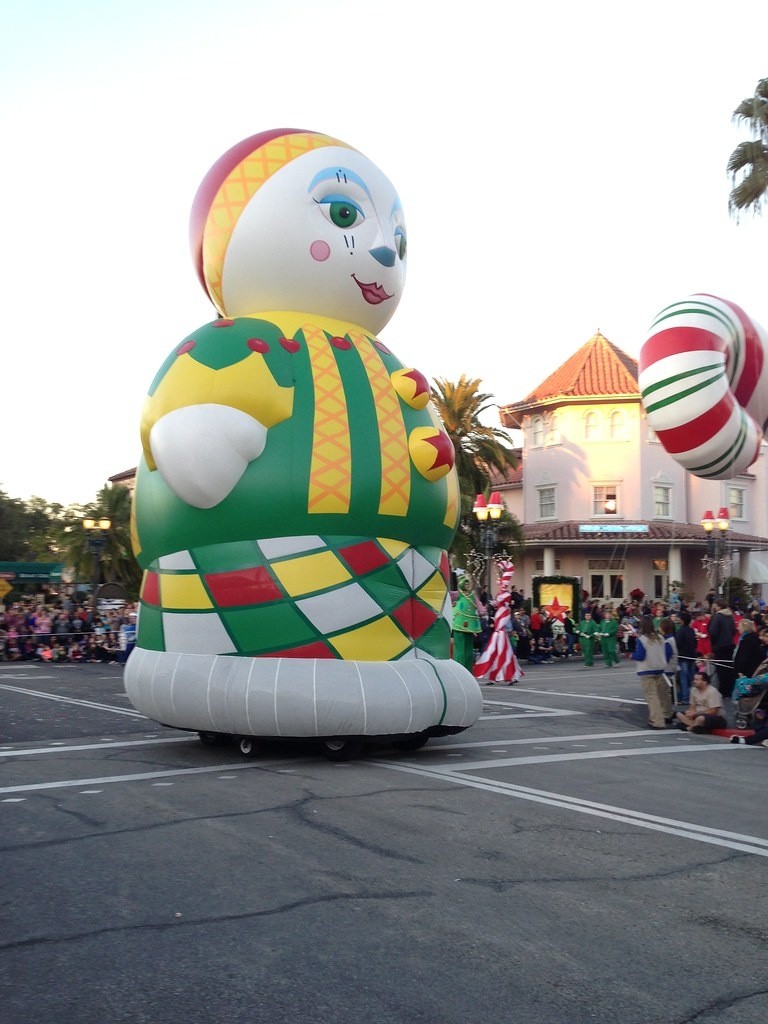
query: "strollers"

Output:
[732,657,768,730]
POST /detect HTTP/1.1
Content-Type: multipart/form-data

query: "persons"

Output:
[0,590,138,665]
[452,581,482,673]
[483,584,768,745]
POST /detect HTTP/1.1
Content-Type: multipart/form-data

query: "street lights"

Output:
[699,507,735,599]
[81,514,112,595]
[463,491,514,600]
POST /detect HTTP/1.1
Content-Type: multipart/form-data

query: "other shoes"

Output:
[677,723,687,731]
[648,725,661,729]
[666,719,672,723]
[730,735,745,743]
[694,726,712,734]
